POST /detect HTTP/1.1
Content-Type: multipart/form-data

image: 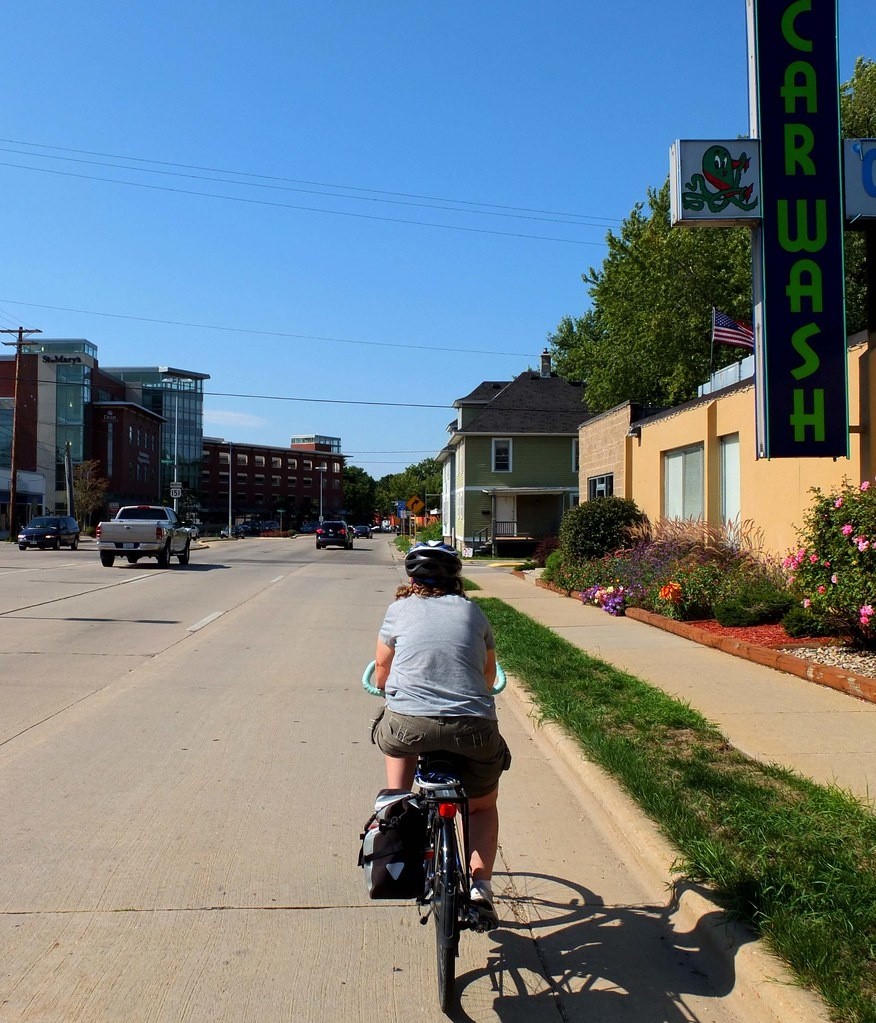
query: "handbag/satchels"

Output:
[358,789,430,901]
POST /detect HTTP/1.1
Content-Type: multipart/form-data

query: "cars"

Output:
[219,518,279,540]
[314,521,354,550]
[17,515,81,551]
[354,525,373,539]
[185,526,201,540]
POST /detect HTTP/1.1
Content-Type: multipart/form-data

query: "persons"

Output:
[368,539,512,933]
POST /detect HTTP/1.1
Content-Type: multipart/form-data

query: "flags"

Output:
[712,307,756,350]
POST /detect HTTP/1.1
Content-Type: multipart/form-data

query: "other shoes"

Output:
[470,890,500,932]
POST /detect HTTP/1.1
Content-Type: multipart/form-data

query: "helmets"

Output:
[405,540,463,582]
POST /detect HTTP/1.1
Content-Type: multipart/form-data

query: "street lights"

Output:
[221,440,234,539]
[314,466,328,524]
[161,377,194,517]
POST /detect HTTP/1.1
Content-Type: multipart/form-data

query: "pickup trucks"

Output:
[95,504,192,569]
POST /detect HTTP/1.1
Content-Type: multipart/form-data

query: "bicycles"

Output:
[359,655,508,1011]
